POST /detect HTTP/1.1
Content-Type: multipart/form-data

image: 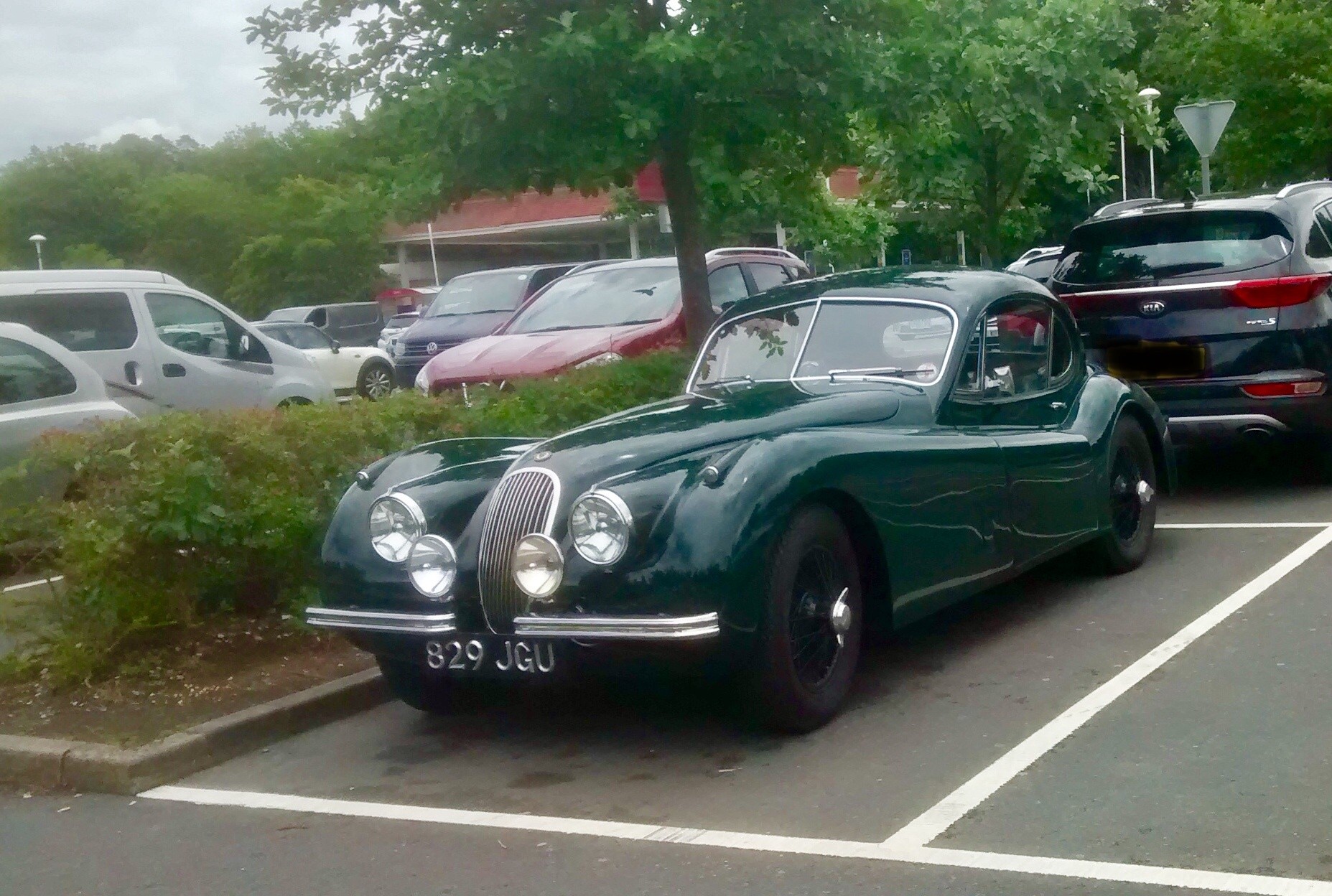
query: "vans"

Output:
[391,261,590,395]
[0,269,339,426]
[264,301,387,351]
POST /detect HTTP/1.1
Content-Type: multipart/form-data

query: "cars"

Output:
[377,310,421,352]
[295,255,1179,732]
[882,244,1068,382]
[0,322,143,467]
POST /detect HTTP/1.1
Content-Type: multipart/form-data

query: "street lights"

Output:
[29,233,49,275]
[1133,85,1162,198]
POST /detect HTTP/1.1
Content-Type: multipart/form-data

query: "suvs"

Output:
[150,320,396,406]
[411,245,817,397]
[1042,179,1332,479]
